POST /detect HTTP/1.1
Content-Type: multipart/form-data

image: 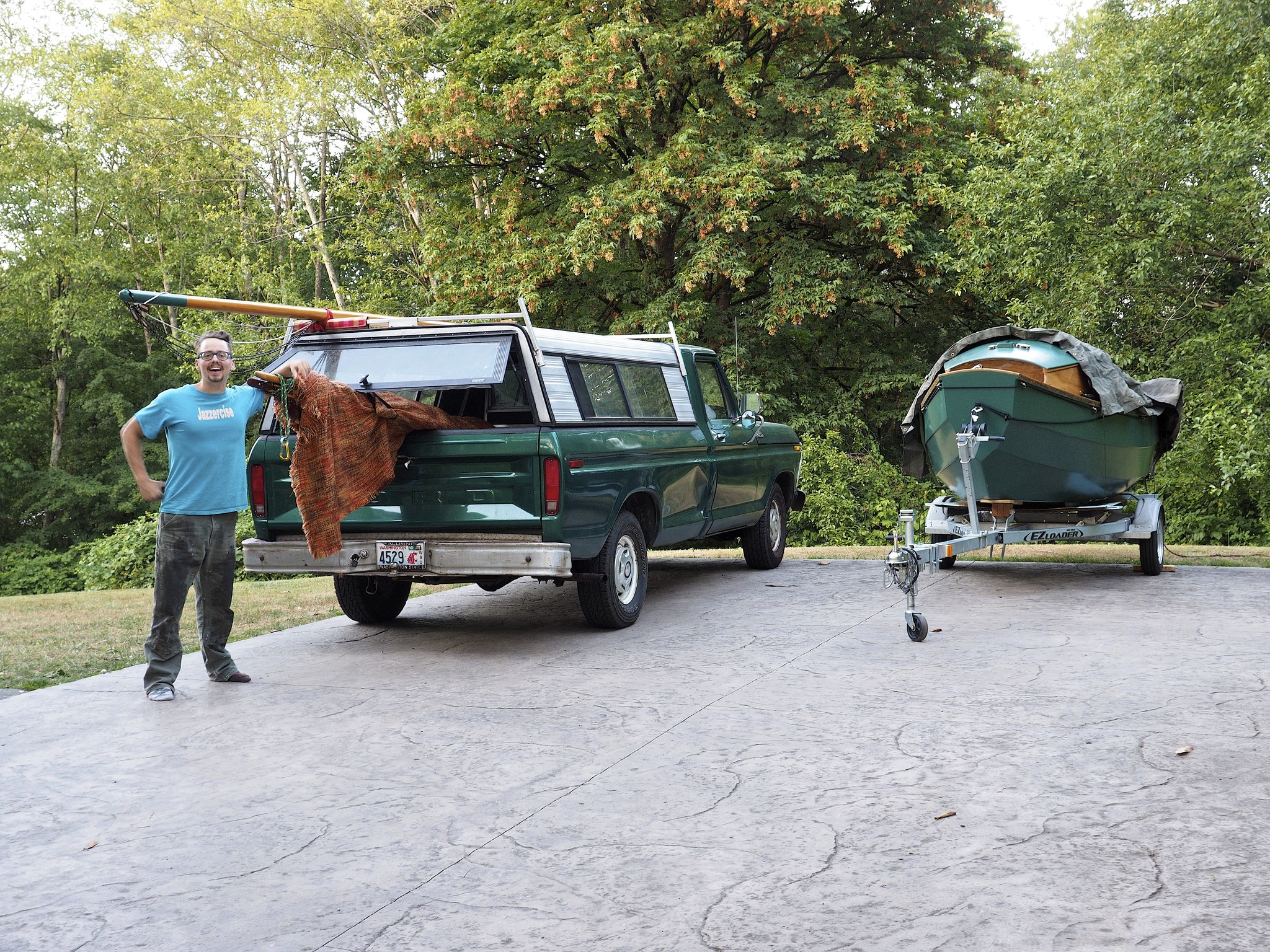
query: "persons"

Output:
[120,330,310,701]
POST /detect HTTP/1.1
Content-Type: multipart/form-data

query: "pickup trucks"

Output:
[240,297,805,624]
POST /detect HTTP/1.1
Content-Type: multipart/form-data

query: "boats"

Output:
[918,338,1162,517]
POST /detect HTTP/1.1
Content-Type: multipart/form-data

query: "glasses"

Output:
[197,351,233,360]
